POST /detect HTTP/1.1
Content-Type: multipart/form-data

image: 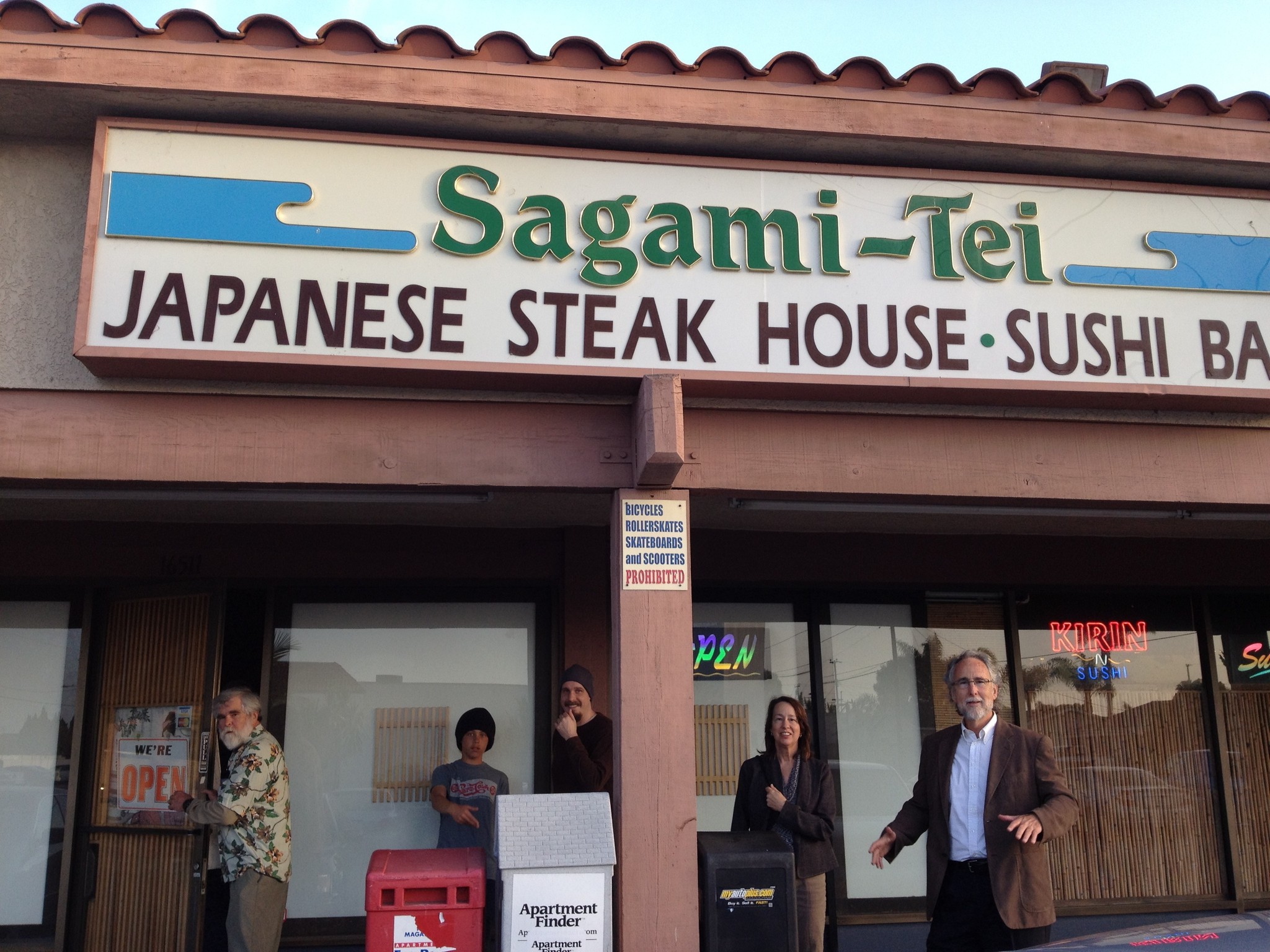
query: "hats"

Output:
[559,665,593,700]
[455,707,495,752]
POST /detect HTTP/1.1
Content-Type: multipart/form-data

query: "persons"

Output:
[429,707,510,952]
[167,687,292,952]
[539,663,613,795]
[731,696,839,952]
[869,646,1079,952]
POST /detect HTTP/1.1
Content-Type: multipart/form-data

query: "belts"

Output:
[950,860,986,873]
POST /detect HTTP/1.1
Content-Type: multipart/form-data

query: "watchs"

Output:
[183,799,194,812]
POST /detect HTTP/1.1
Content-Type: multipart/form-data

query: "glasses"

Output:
[953,679,991,690]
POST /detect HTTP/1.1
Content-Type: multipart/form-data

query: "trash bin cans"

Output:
[699,836,800,952]
[493,791,617,952]
[365,847,486,952]
